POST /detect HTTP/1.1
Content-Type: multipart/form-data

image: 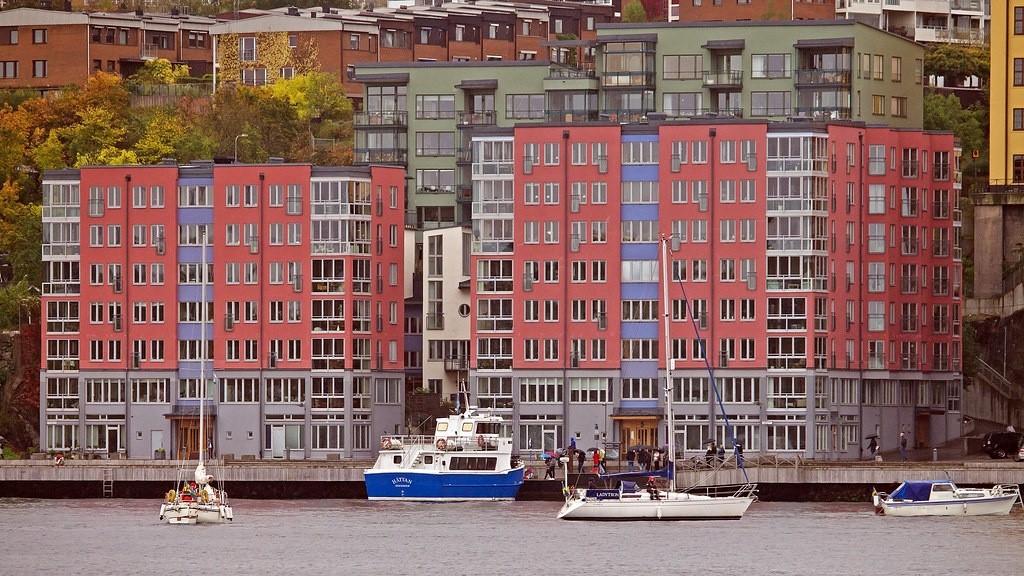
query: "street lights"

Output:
[601,402,606,476]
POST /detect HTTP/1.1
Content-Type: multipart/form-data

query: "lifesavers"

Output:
[168,490,175,502]
[478,435,484,446]
[991,486,1003,496]
[55,454,64,466]
[436,438,446,449]
[200,490,208,502]
[524,467,535,479]
[381,438,392,449]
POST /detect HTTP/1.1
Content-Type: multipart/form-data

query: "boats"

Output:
[872,477,1024,519]
[361,382,533,503]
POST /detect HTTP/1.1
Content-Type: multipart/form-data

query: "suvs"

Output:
[980,432,1024,459]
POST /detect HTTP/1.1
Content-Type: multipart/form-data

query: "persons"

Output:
[544,457,556,480]
[54,453,64,465]
[592,448,607,475]
[626,449,669,472]
[900,433,908,462]
[577,452,585,474]
[705,440,744,468]
[0,441,5,459]
[867,438,877,459]
[207,437,213,460]
[646,477,662,500]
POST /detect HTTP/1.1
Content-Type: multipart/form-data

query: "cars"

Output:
[1016,443,1024,461]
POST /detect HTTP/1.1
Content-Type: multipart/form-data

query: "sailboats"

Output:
[555,231,759,524]
[158,231,234,525]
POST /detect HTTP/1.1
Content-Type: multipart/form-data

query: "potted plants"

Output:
[154,448,165,459]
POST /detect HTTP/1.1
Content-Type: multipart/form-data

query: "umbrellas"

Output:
[626,445,668,451]
[703,438,715,443]
[574,448,586,454]
[587,447,599,451]
[0,436,5,440]
[865,434,879,438]
[539,452,559,458]
